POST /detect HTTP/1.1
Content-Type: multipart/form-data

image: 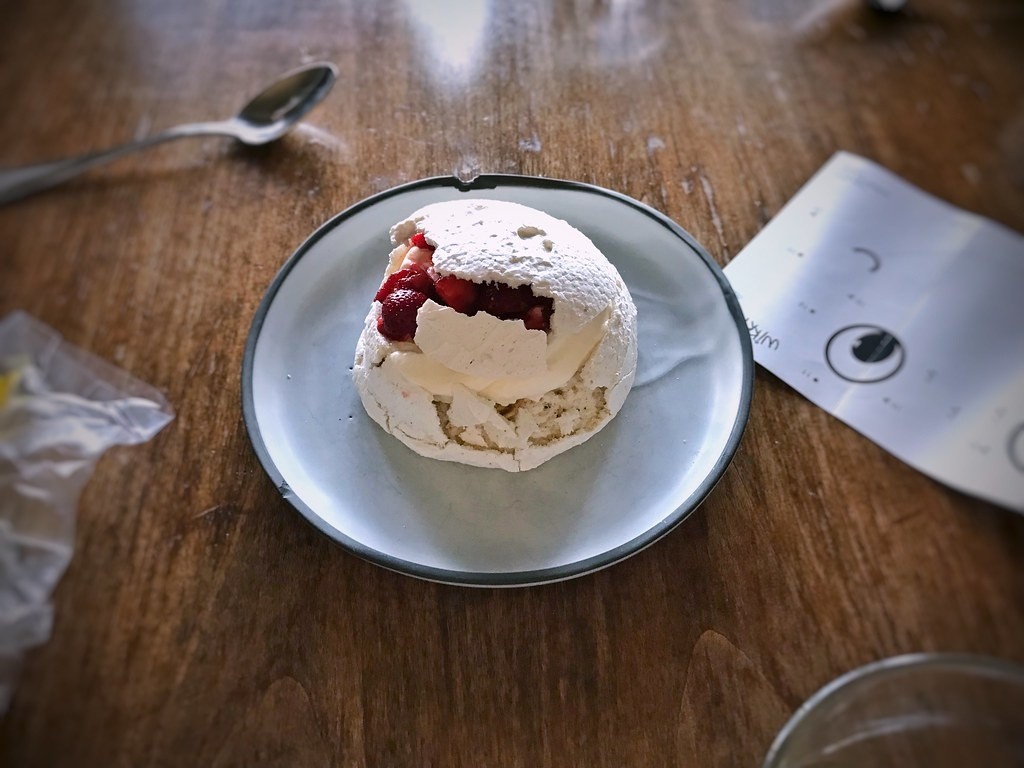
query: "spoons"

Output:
[0,65,339,207]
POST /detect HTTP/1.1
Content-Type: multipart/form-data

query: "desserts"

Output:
[352,199,637,472]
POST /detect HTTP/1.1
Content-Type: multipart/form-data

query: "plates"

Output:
[240,173,755,590]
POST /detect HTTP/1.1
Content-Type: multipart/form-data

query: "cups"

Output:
[762,652,1024,768]
